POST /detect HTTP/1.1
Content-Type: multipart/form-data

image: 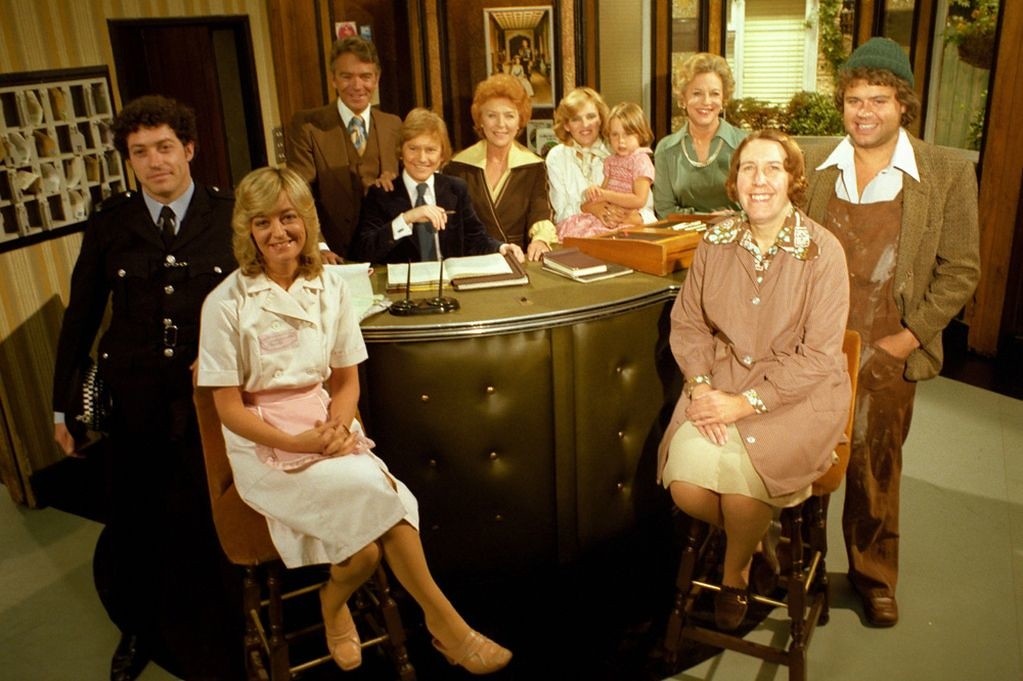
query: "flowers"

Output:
[946,0,999,41]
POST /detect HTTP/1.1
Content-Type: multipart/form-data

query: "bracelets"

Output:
[343,425,351,435]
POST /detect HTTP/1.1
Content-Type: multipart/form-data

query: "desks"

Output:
[357,242,689,623]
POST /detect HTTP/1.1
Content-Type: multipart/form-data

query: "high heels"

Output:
[319,590,362,672]
[429,631,512,674]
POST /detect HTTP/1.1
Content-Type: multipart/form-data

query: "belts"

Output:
[123,326,196,346]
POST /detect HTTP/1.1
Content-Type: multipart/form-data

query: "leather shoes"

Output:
[858,580,897,626]
[110,629,153,681]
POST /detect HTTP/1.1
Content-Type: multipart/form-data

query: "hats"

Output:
[64,355,114,445]
[512,55,520,64]
[845,37,914,87]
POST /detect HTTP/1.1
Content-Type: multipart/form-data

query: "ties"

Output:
[416,183,437,261]
[351,116,365,156]
[160,207,175,248]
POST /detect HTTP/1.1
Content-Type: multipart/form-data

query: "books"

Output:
[386,253,530,291]
[542,247,634,284]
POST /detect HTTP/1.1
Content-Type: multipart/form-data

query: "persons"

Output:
[198,165,515,676]
[511,54,534,97]
[644,130,849,631]
[52,96,240,681]
[517,39,532,83]
[283,37,752,275]
[801,36,980,624]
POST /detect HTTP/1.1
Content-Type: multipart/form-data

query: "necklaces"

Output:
[680,135,723,167]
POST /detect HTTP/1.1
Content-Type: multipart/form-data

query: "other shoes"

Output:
[715,585,749,631]
[751,521,782,596]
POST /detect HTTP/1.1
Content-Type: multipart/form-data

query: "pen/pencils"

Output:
[441,210,456,213]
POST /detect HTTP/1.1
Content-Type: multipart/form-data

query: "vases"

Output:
[956,33,997,69]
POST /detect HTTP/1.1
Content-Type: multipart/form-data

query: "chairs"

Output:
[661,328,861,681]
[191,357,419,681]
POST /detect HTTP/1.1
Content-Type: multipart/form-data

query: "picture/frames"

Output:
[483,4,556,111]
